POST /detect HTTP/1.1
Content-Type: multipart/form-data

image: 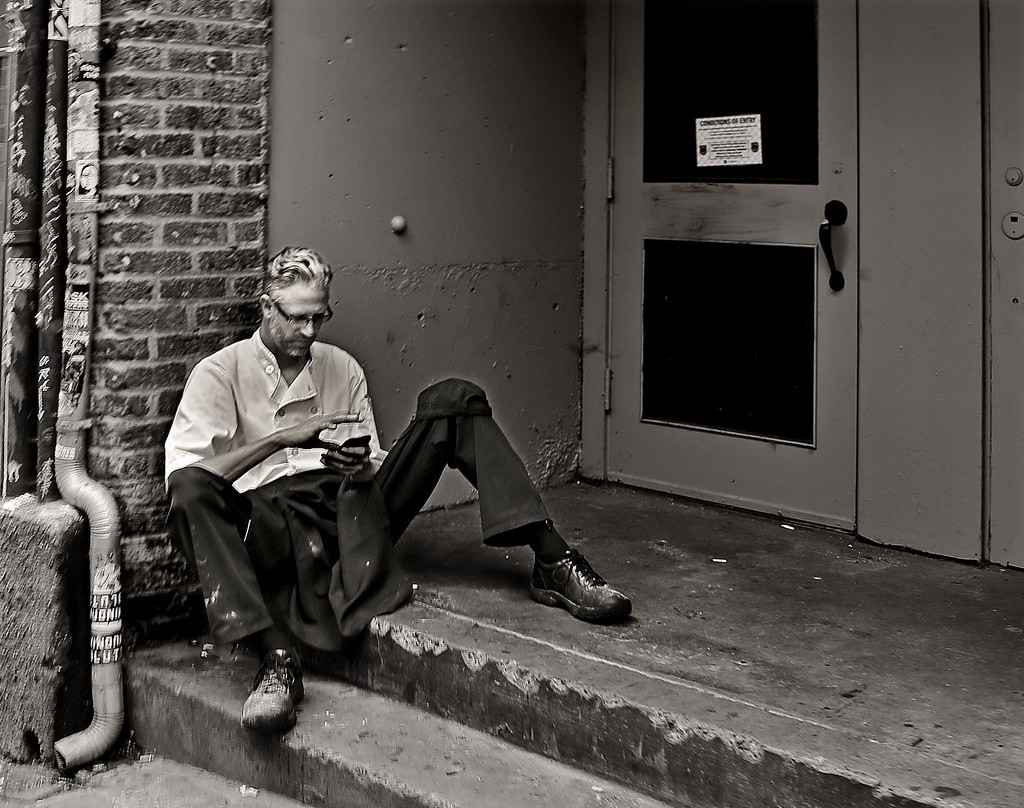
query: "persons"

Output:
[164,247,632,729]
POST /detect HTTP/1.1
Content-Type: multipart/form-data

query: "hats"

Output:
[396,376,493,442]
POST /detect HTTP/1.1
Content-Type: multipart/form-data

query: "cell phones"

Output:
[337,435,371,455]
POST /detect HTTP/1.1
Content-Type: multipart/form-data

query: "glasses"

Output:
[264,291,333,328]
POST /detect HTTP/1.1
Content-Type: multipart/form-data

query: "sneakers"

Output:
[242,647,304,731]
[528,548,632,624]
